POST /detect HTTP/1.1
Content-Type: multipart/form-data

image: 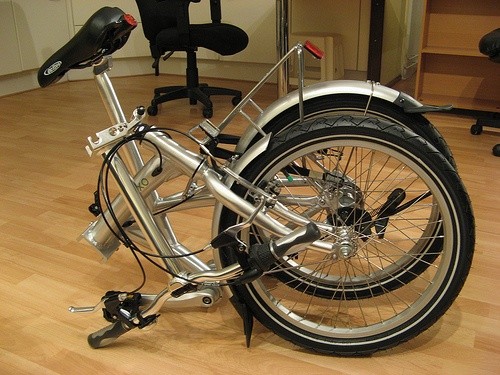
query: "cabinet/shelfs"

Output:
[157,0,279,84]
[0,0,76,97]
[70,0,158,82]
[288,0,423,86]
[414,0,500,113]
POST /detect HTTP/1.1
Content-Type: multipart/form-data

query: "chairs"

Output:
[135,0,249,118]
[470,28,500,157]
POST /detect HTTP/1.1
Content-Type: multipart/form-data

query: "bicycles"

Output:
[34,4,477,360]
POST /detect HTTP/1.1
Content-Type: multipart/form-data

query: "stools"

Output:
[288,32,344,86]
